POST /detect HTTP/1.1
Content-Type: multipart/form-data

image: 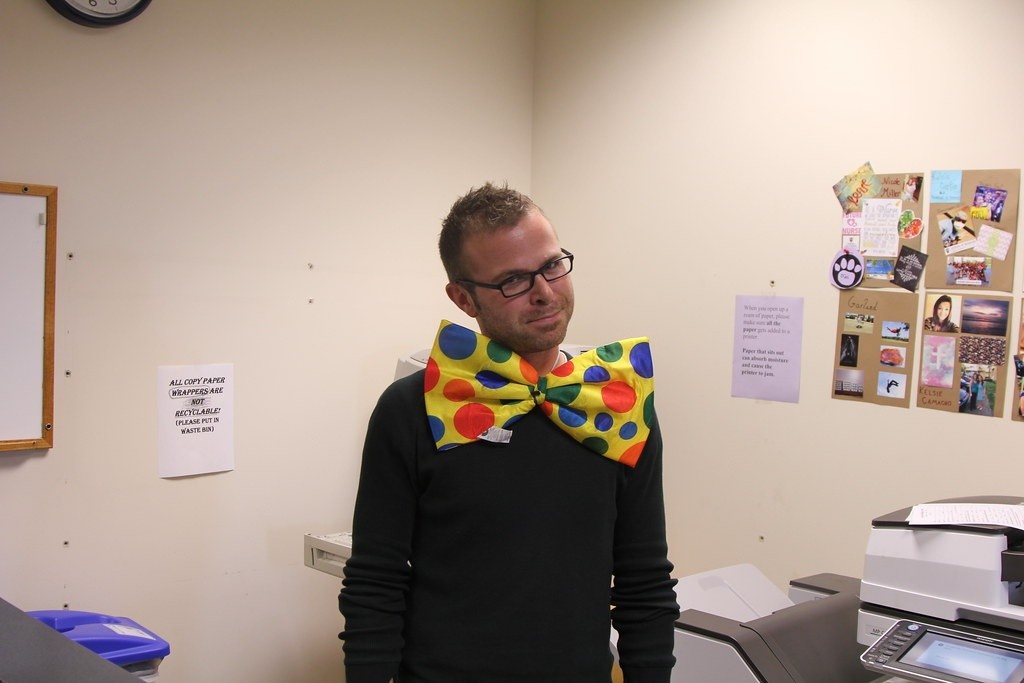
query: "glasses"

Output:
[455,246,574,299]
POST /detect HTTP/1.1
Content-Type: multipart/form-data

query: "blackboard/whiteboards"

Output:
[0,179,58,453]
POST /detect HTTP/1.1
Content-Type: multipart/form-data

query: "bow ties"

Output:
[423,313,654,469]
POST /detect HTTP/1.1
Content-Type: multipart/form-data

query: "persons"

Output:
[971,191,1000,221]
[925,295,959,333]
[904,179,916,197]
[938,211,968,247]
[969,373,978,411]
[335,181,681,682]
[976,374,986,410]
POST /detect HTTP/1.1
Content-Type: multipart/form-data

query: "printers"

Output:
[610,496,1024,683]
